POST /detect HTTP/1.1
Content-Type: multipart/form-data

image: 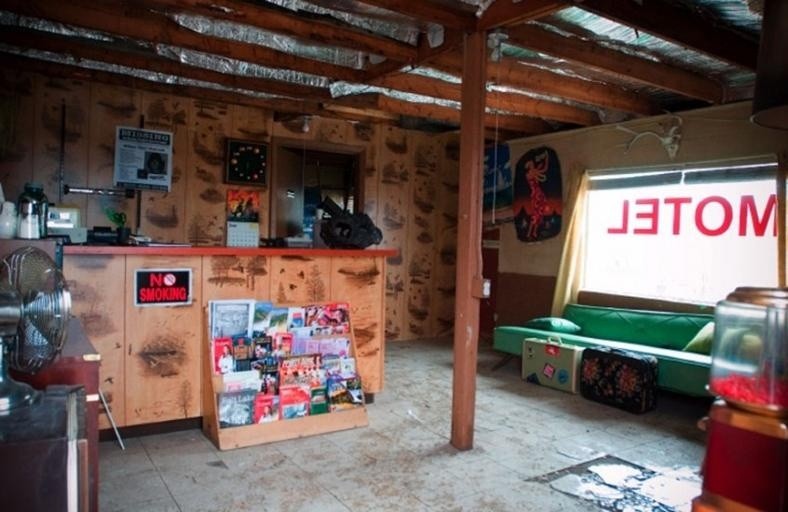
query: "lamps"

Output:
[302,117,310,133]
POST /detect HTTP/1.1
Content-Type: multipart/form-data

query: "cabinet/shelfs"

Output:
[202,301,369,452]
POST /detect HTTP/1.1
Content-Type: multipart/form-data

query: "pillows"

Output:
[524,317,581,333]
[682,322,750,361]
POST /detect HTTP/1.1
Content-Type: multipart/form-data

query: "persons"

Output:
[218,337,267,373]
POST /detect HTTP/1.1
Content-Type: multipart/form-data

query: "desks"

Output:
[10,319,100,512]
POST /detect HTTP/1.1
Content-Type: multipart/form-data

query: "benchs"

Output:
[492,304,765,398]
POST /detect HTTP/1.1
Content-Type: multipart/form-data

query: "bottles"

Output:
[706,285,784,419]
[0,202,18,240]
[18,182,48,239]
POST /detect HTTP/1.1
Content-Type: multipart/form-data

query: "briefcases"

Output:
[580,345,658,414]
[521,335,585,393]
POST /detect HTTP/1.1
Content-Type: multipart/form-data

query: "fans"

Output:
[0,246,71,417]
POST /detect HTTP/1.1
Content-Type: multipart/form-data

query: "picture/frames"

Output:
[224,137,269,188]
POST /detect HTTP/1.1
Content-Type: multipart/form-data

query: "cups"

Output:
[116,227,132,244]
